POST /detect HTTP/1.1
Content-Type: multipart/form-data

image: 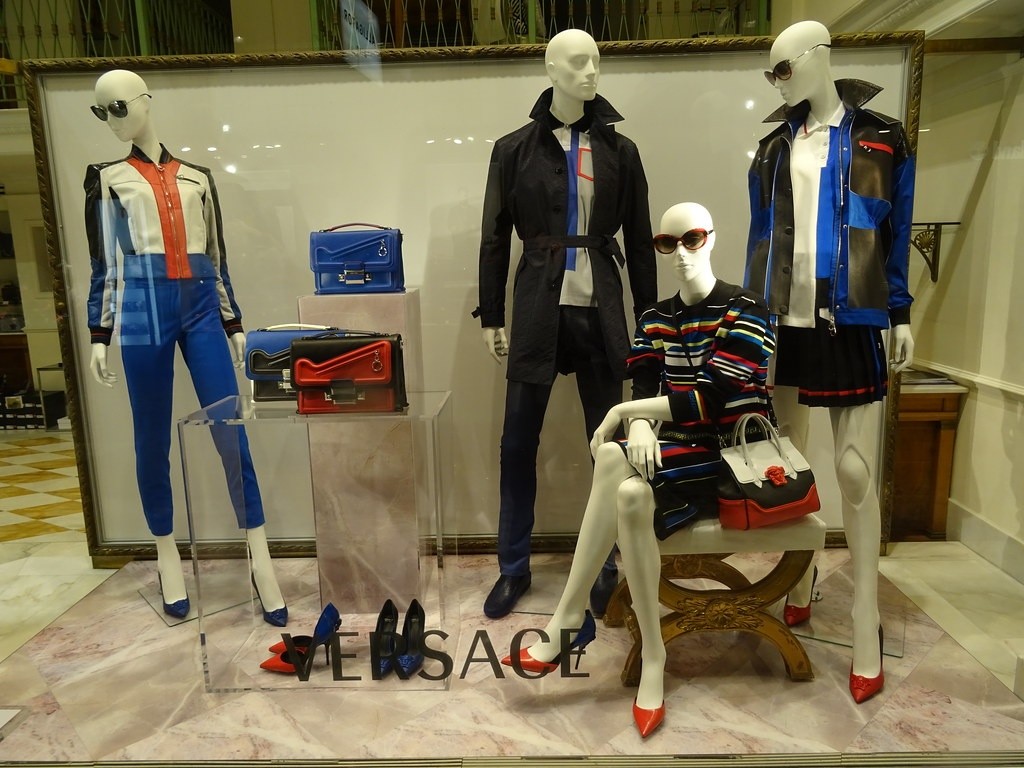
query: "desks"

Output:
[886,367,970,542]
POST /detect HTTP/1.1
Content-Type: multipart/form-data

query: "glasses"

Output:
[764,44,834,88]
[91,94,152,122]
[654,228,714,255]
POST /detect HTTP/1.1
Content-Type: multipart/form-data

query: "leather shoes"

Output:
[484,571,532,620]
[590,566,618,619]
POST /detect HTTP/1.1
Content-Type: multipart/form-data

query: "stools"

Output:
[601,512,827,688]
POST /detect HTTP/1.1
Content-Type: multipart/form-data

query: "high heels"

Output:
[157,570,190,619]
[632,695,665,738]
[783,565,819,627]
[849,623,883,704]
[259,613,335,675]
[396,599,426,680]
[371,599,399,680]
[499,610,597,674]
[249,570,288,628]
[269,603,341,666]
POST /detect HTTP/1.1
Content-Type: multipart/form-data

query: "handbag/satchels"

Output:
[717,413,821,530]
[291,330,409,414]
[309,222,407,295]
[244,323,349,402]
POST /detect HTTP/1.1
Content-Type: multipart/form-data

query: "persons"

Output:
[83,70,288,626]
[742,19,915,704]
[471,29,660,619]
[502,202,775,738]
